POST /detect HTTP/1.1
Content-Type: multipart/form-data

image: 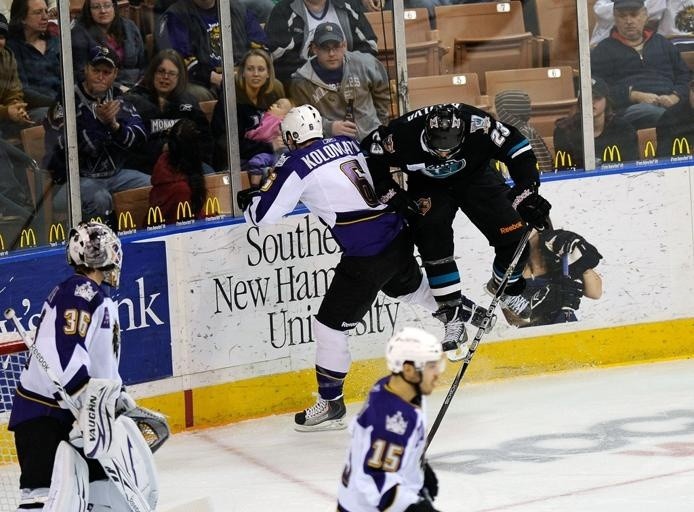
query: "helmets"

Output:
[277,103,326,146]
[65,221,125,289]
[424,99,466,160]
[382,326,448,379]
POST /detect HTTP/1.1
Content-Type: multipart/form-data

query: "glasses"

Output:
[156,69,181,80]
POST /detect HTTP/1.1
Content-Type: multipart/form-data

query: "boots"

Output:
[293,391,348,426]
[485,265,534,320]
[431,302,470,352]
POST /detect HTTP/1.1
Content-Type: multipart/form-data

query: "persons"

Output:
[234,102,466,427]
[331,326,444,512]
[0,1,397,259]
[483,191,607,328]
[354,101,545,351]
[552,73,642,172]
[4,220,133,511]
[579,1,693,159]
[493,88,551,170]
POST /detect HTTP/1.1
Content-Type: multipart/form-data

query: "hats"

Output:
[85,44,120,69]
[612,0,646,10]
[314,21,346,47]
[592,74,609,99]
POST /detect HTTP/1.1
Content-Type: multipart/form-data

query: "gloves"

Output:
[505,182,552,231]
[236,185,261,212]
